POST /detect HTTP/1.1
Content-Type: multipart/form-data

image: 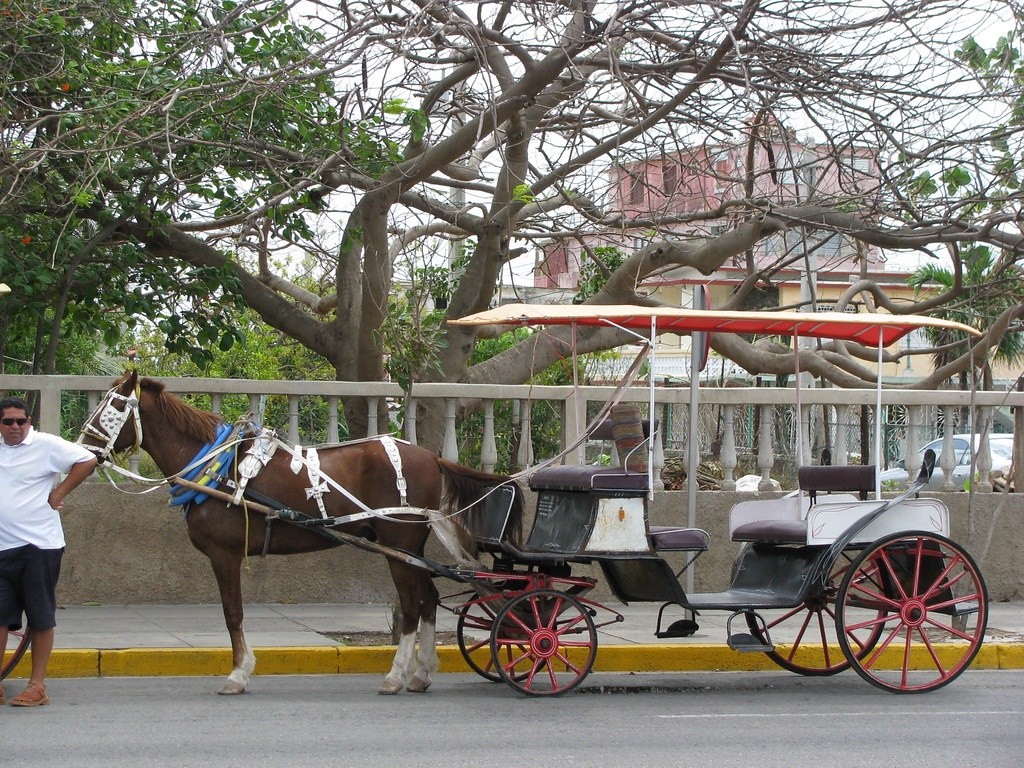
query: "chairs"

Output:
[530,419,658,490]
[731,465,875,543]
[649,525,712,549]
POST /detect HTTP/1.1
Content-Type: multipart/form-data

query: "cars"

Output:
[879,432,1014,491]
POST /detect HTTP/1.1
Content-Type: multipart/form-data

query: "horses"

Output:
[71,366,525,696]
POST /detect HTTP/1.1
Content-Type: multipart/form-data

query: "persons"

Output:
[0,396,98,707]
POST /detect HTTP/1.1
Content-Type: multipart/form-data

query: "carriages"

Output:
[75,302,990,696]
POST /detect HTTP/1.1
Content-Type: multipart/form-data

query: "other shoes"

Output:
[0,684,6,705]
[9,684,49,706]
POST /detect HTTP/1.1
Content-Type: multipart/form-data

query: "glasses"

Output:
[1,418,29,426]
